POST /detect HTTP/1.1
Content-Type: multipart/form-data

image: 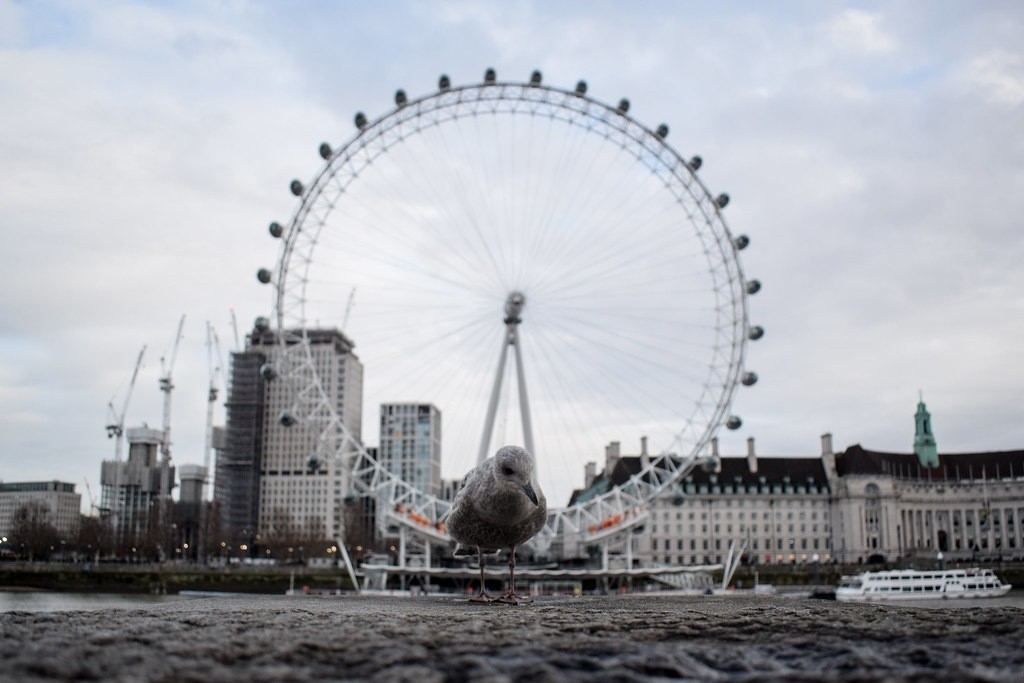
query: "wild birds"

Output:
[445,445,548,604]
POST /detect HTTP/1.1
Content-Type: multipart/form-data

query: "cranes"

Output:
[105,306,241,565]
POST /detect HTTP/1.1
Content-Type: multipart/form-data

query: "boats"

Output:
[835,566,1012,602]
[347,561,726,599]
